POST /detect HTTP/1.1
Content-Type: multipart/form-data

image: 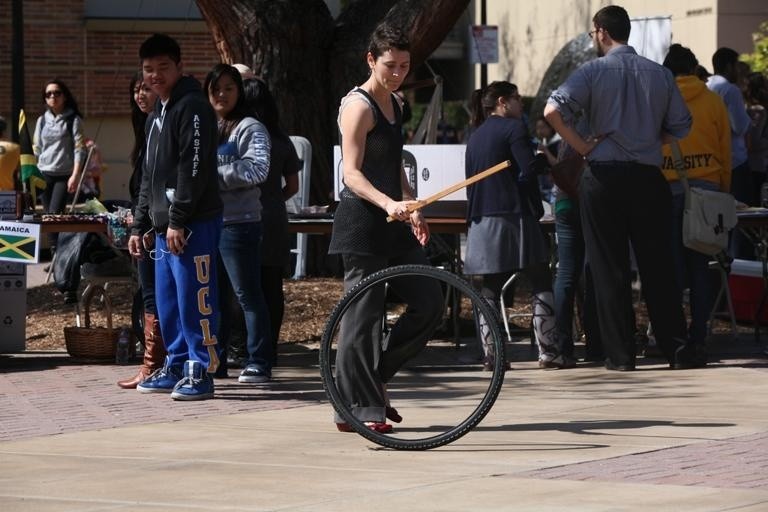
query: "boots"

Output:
[118,312,168,388]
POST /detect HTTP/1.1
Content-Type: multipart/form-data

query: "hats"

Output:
[229,62,269,87]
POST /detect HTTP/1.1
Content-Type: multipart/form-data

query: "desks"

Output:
[20,215,137,235]
[469,205,768,343]
[286,218,468,363]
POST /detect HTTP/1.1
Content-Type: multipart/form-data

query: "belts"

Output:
[586,160,661,169]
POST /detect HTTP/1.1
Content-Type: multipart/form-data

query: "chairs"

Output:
[279,136,313,280]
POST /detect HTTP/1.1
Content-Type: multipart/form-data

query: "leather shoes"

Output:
[670,361,707,370]
[605,358,637,373]
[386,405,403,424]
[335,420,394,434]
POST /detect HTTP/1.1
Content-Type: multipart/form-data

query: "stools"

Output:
[77,263,140,332]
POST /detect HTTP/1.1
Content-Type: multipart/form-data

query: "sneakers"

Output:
[135,356,188,394]
[227,359,248,368]
[541,357,578,370]
[483,357,512,372]
[171,360,215,401]
[238,367,270,383]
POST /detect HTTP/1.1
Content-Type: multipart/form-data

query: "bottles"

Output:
[116,322,132,366]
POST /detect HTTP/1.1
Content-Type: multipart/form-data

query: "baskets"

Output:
[64,285,132,360]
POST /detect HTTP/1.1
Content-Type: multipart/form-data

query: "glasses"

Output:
[44,89,63,99]
[506,93,523,103]
[587,28,602,37]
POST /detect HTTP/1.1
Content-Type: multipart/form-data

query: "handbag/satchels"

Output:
[668,134,739,258]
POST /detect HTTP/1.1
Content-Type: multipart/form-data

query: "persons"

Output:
[661,43,733,340]
[0,115,27,221]
[242,79,302,358]
[533,114,563,202]
[118,70,163,390]
[705,47,753,199]
[328,21,445,434]
[544,3,705,369]
[32,79,87,271]
[745,71,767,206]
[203,61,273,381]
[128,34,221,397]
[553,137,606,368]
[463,81,565,368]
